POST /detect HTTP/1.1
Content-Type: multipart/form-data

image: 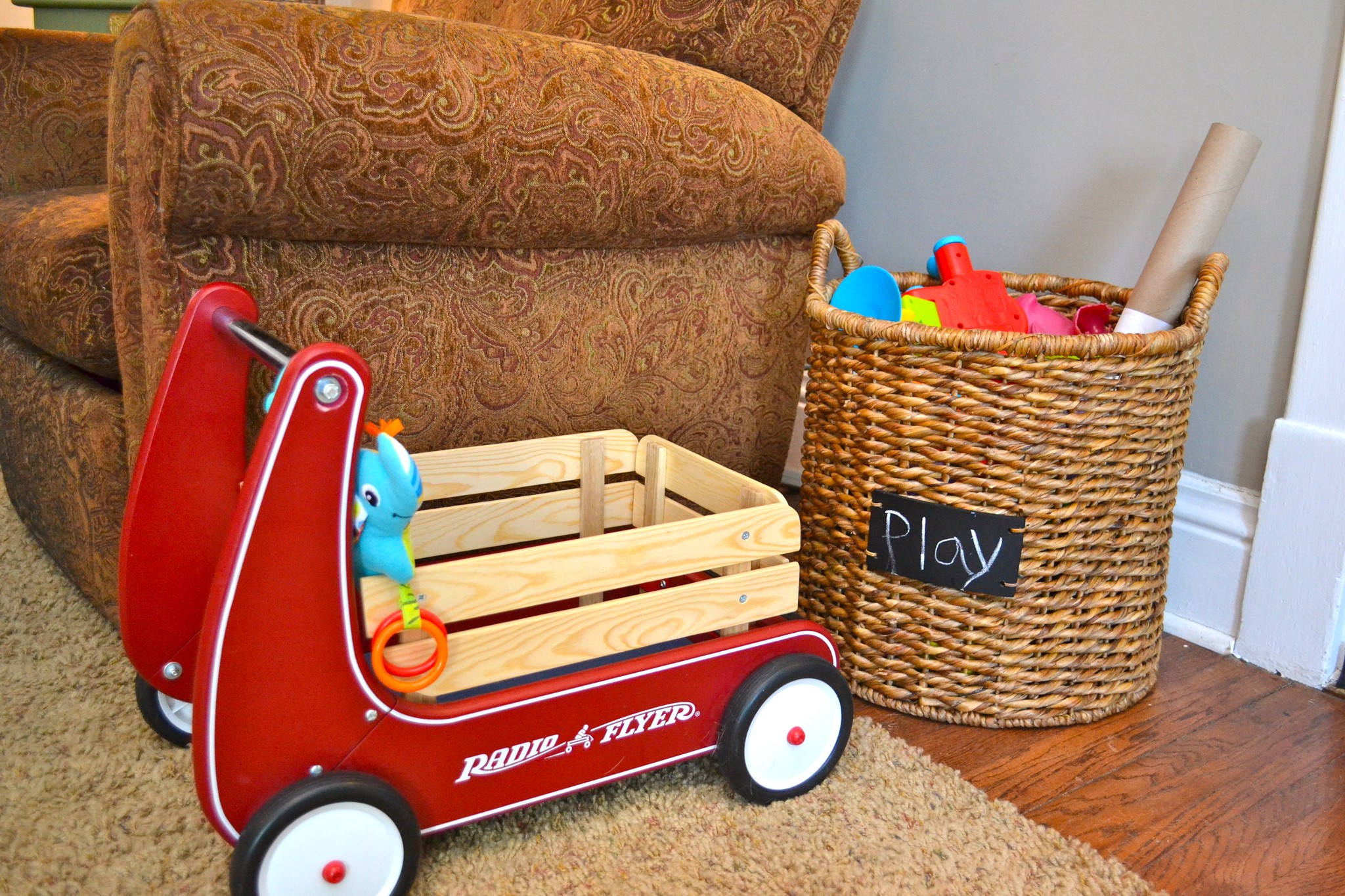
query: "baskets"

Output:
[790,219,1229,728]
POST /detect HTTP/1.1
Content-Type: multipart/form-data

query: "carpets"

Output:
[0,472,1172,896]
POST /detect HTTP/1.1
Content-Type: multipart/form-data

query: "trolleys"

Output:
[114,280,853,896]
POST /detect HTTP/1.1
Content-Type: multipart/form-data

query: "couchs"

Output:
[0,0,862,626]
